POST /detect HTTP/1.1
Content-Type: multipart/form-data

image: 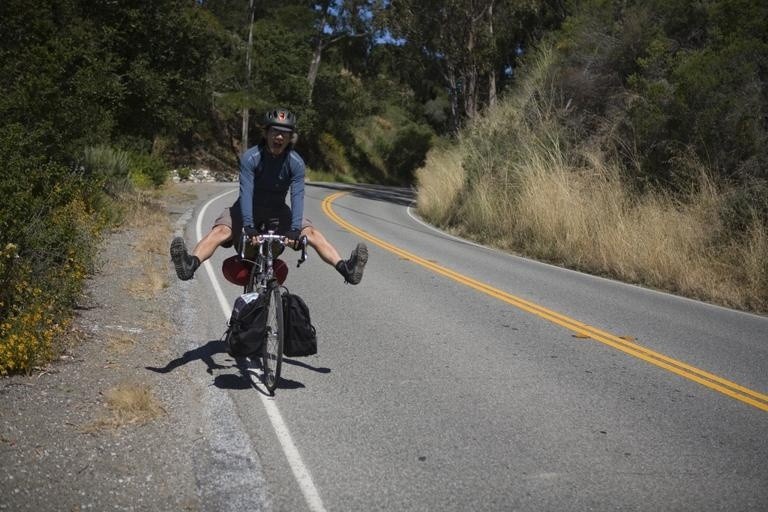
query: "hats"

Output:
[271,125,293,132]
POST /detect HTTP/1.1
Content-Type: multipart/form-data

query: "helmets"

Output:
[263,108,297,129]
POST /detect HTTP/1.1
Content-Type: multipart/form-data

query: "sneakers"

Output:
[170,236,194,280]
[342,242,368,284]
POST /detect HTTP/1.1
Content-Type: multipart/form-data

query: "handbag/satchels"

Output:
[224,291,316,357]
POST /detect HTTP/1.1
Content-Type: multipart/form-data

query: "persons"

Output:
[170,109,368,285]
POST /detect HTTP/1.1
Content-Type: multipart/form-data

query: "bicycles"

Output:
[233,225,309,394]
